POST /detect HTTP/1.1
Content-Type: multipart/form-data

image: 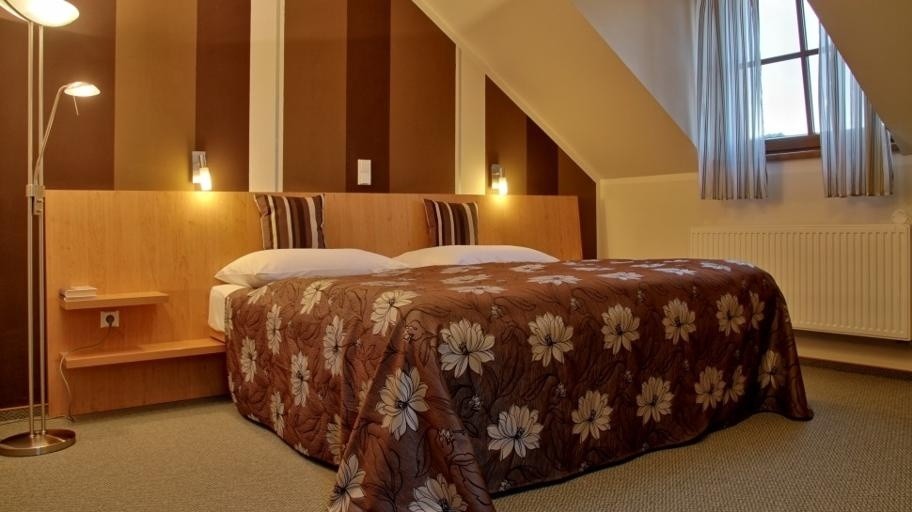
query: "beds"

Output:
[203,251,819,511]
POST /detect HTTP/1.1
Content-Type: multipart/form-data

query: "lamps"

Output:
[489,161,509,198]
[0,0,107,459]
[190,147,214,191]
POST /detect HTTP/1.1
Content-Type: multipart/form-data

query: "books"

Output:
[59,285,98,298]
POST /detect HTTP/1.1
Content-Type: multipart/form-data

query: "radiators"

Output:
[688,221,911,380]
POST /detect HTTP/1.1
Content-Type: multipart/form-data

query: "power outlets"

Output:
[99,310,121,330]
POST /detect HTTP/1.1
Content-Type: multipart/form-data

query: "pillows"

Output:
[251,191,330,250]
[421,198,481,249]
[209,245,411,290]
[387,242,560,270]
